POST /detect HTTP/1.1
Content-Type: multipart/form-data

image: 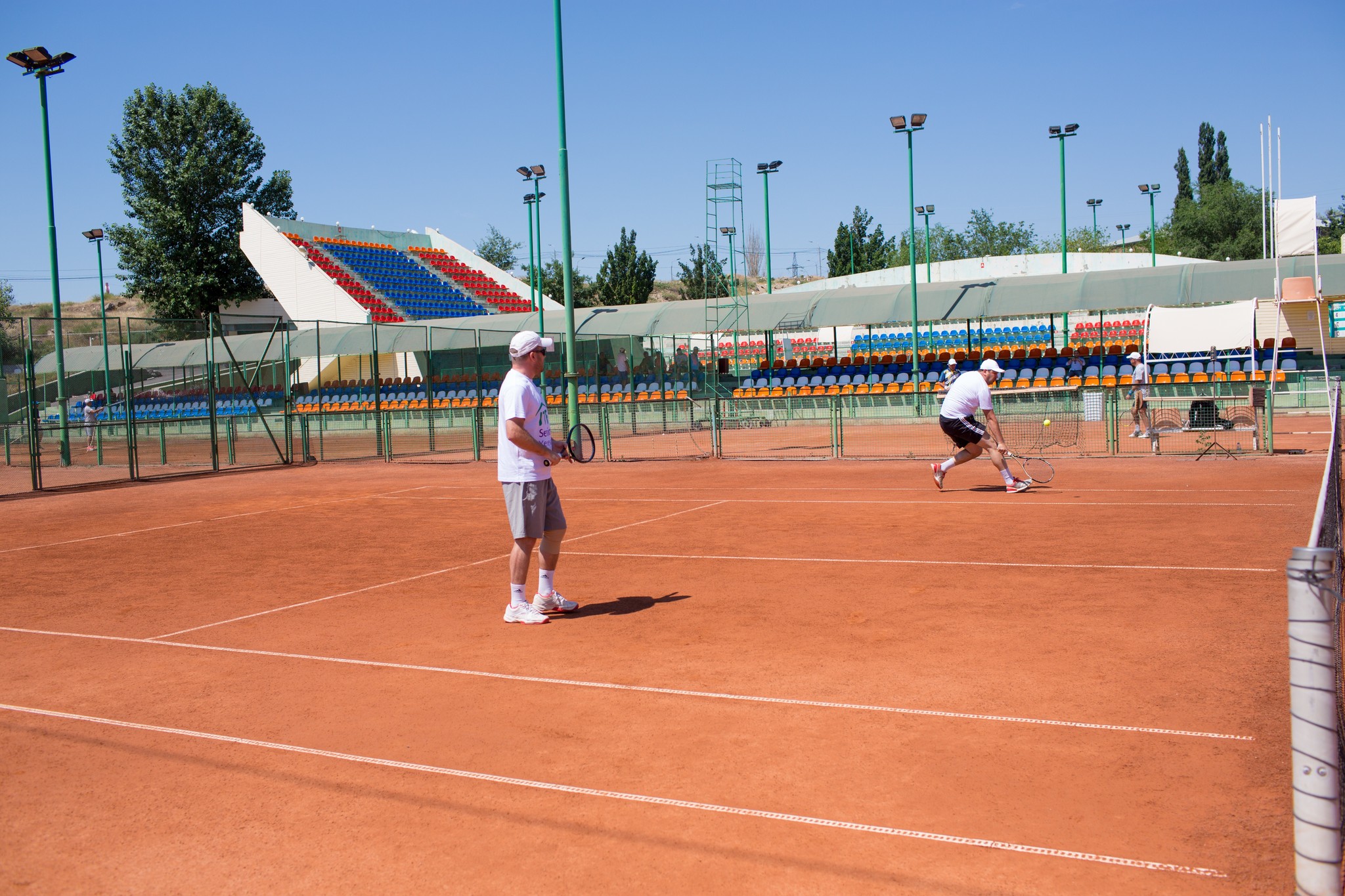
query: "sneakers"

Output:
[87,446,94,451]
[1129,429,1143,437]
[697,389,702,392]
[671,377,678,380]
[504,600,549,625]
[931,463,947,489]
[91,446,96,450]
[39,447,44,450]
[1138,432,1150,438]
[532,590,579,612]
[1006,477,1033,493]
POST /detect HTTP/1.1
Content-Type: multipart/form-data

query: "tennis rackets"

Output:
[1006,452,1054,484]
[1120,407,1135,427]
[543,423,596,467]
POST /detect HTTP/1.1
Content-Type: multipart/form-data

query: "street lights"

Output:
[806,258,826,276]
[808,241,821,277]
[1116,224,1131,253]
[1047,123,1080,347]
[1086,199,1103,253]
[671,258,681,281]
[889,113,928,417]
[1137,183,1161,267]
[914,204,935,353]
[577,257,586,277]
[694,236,706,272]
[548,244,556,274]
[756,160,784,368]
[5,45,79,467]
[516,165,547,407]
[849,228,856,275]
[719,226,739,376]
[522,191,546,312]
[736,262,744,274]
[81,228,114,436]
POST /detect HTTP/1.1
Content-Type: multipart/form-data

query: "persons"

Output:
[496,330,579,625]
[930,358,1033,494]
[672,348,688,382]
[1066,350,1086,382]
[637,351,668,375]
[1126,352,1153,439]
[938,358,962,391]
[595,352,614,375]
[614,347,629,389]
[688,346,702,392]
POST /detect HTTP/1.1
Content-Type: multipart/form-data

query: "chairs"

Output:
[41,373,672,425]
[672,302,1297,400]
[279,231,545,322]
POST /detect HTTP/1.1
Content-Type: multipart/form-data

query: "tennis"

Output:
[1044,419,1051,426]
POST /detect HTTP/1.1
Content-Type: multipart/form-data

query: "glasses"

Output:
[528,348,546,356]
[1129,358,1133,361]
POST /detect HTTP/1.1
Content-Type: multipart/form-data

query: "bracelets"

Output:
[1071,358,1072,360]
[1128,389,1134,396]
[1079,359,1080,362]
[944,386,949,390]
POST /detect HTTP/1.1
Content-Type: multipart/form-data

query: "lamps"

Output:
[1177,251,1182,257]
[371,225,439,233]
[1226,257,1230,262]
[510,272,513,276]
[548,294,552,299]
[251,203,370,314]
[797,280,800,285]
[752,291,755,295]
[1078,248,1082,252]
[1129,248,1133,253]
[472,250,476,255]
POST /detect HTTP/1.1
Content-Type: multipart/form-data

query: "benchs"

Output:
[1150,405,1258,455]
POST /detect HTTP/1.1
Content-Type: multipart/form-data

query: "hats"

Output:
[509,331,552,357]
[676,348,681,351]
[1126,352,1141,359]
[619,348,626,352]
[948,359,957,365]
[85,399,93,404]
[693,347,700,350]
[33,401,40,404]
[981,359,1006,373]
